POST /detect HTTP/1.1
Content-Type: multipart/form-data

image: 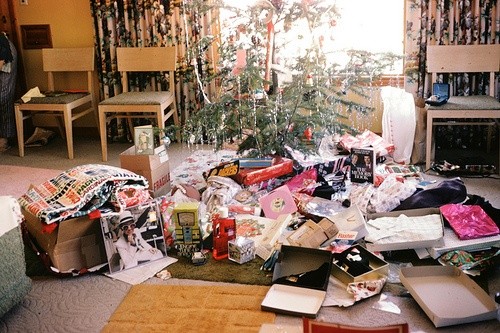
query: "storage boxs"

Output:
[120,145,172,198]
[20,206,108,275]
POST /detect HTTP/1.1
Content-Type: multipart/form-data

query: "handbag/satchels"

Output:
[391,179,467,212]
[220,154,292,187]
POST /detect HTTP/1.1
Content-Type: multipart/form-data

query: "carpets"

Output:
[188,333,188,333]
[160,249,275,285]
[100,285,276,333]
[0,165,133,333]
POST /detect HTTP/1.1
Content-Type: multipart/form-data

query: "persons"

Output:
[0,31,18,153]
[351,154,359,170]
[361,155,373,176]
[116,211,164,271]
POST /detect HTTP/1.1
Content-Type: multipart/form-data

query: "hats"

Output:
[117,210,136,228]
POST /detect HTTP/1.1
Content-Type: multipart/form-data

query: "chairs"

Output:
[97,46,181,162]
[14,46,100,160]
[426,44,500,175]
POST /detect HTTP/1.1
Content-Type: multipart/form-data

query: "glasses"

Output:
[121,223,136,232]
